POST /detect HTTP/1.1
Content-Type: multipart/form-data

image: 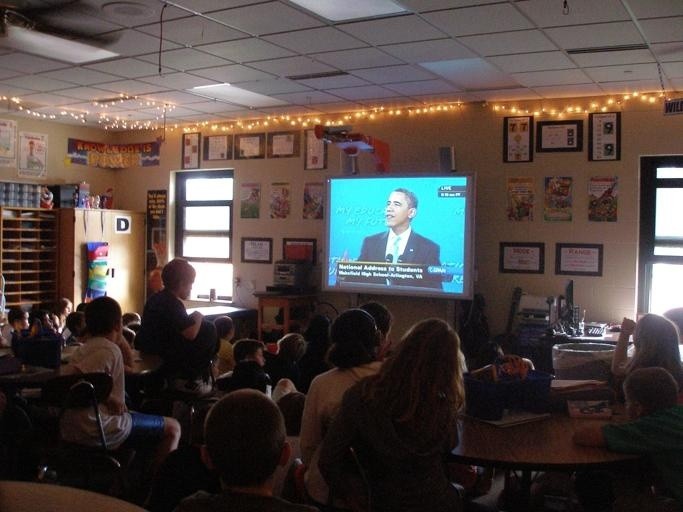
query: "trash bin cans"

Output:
[552,343,617,380]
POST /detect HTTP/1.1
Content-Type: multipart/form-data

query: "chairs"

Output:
[40,371,128,500]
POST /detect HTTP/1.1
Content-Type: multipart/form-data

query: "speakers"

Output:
[339,150,355,172]
[438,145,457,172]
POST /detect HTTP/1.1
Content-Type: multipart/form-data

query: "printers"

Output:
[517,294,565,327]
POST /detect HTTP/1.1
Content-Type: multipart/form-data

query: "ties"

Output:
[393,235,402,263]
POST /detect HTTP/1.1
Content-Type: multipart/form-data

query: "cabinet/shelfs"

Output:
[253,290,317,342]
[60,207,149,316]
[1,182,61,325]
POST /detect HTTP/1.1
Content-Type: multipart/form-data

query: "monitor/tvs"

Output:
[321,171,478,330]
[565,280,581,336]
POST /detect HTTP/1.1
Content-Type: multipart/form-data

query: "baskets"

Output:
[10,328,65,369]
[463,364,556,421]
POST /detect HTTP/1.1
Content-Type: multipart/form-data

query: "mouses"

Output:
[610,326,620,332]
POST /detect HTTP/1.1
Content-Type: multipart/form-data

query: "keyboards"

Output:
[587,326,604,336]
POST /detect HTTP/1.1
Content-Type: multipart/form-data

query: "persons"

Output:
[134,259,204,365]
[298,308,385,512]
[55,297,182,507]
[571,366,682,512]
[317,317,465,511]
[344,188,443,289]
[1,296,683,467]
[173,389,318,511]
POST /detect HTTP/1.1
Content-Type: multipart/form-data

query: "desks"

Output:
[452,414,640,511]
[186,304,258,342]
[1,481,150,512]
[556,332,634,346]
